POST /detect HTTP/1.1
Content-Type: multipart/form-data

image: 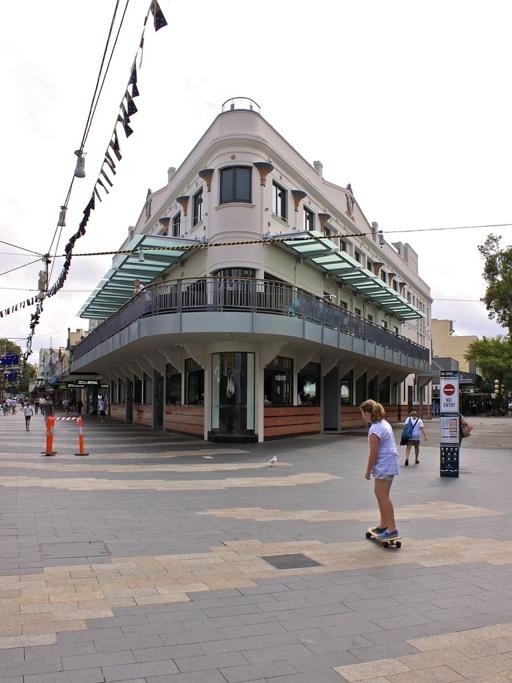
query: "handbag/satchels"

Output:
[402,422,412,440]
[461,422,473,438]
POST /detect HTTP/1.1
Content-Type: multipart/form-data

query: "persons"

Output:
[86,396,107,425]
[359,399,400,540]
[144,188,152,224]
[80,323,98,341]
[432,399,440,416]
[403,411,428,466]
[134,279,153,312]
[460,413,472,452]
[0,390,84,432]
[463,395,512,417]
[344,183,356,223]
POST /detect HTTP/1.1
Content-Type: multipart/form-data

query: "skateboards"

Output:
[366,527,401,548]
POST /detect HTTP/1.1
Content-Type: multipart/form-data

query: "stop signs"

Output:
[441,377,460,413]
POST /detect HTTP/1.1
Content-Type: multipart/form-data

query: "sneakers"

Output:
[415,460,420,463]
[378,529,400,541]
[405,459,408,465]
[372,526,387,534]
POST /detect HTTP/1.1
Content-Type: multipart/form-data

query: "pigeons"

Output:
[269,455,278,464]
